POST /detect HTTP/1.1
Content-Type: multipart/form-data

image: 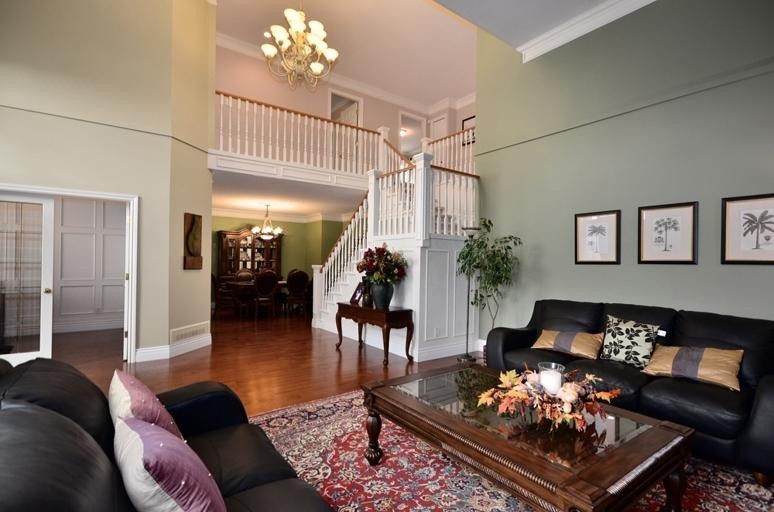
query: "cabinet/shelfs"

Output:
[217,229,283,277]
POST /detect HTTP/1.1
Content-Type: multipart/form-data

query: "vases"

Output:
[371,280,394,312]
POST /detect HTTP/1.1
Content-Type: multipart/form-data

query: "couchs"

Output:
[0,357,332,511]
[485,300,773,488]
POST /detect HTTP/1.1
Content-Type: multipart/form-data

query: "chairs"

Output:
[212,274,237,320]
[234,269,254,281]
[276,270,308,324]
[248,269,278,323]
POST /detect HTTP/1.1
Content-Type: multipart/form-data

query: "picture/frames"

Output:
[637,201,699,264]
[184,212,203,270]
[720,194,774,265]
[462,116,476,146]
[349,282,366,306]
[573,210,621,266]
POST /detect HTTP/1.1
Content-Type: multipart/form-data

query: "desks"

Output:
[335,301,414,367]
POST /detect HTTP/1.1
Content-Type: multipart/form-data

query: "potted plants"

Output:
[455,217,522,367]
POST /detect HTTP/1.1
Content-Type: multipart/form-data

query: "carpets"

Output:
[244,389,773,511]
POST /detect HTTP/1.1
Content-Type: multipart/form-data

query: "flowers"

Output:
[357,242,407,285]
[477,369,622,431]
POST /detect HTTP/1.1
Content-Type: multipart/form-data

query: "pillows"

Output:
[599,315,659,371]
[531,328,604,360]
[113,417,228,512]
[641,343,745,391]
[108,368,188,441]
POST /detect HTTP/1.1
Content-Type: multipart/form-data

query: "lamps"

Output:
[251,205,284,244]
[457,227,481,364]
[260,0,339,90]
